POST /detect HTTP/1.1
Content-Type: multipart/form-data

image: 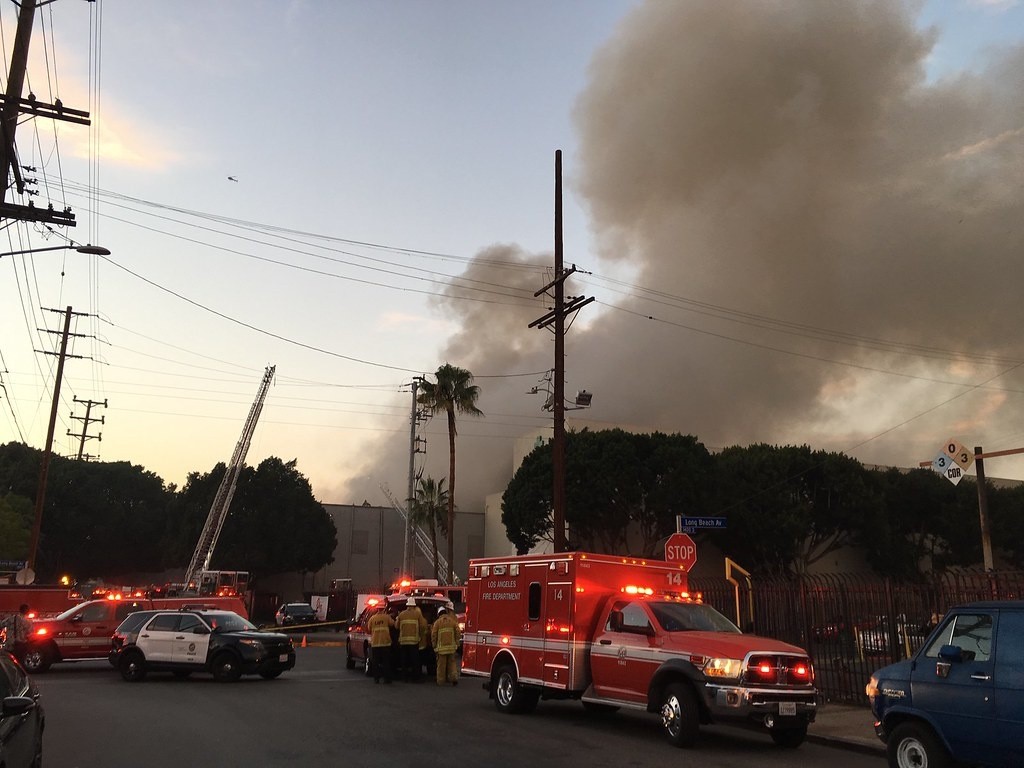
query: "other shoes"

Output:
[453,681,458,686]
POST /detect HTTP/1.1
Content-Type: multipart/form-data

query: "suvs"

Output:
[109,602,297,684]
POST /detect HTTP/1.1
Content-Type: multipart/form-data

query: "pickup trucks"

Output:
[24,595,250,675]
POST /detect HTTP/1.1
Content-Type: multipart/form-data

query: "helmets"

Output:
[445,602,454,611]
[376,603,385,608]
[437,606,445,614]
[406,597,416,606]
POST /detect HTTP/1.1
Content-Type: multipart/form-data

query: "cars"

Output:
[0,627,47,768]
[345,595,451,676]
[274,601,319,634]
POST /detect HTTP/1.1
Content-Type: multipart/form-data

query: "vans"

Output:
[864,598,1024,768]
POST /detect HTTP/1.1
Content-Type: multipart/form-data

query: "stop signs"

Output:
[664,533,696,573]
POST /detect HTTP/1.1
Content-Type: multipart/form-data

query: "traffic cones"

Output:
[300,635,307,648]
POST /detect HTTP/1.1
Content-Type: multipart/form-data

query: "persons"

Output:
[394,597,461,686]
[0,603,34,662]
[368,603,395,684]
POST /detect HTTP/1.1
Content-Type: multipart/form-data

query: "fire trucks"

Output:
[457,549,820,751]
[150,363,277,609]
[377,482,468,652]
